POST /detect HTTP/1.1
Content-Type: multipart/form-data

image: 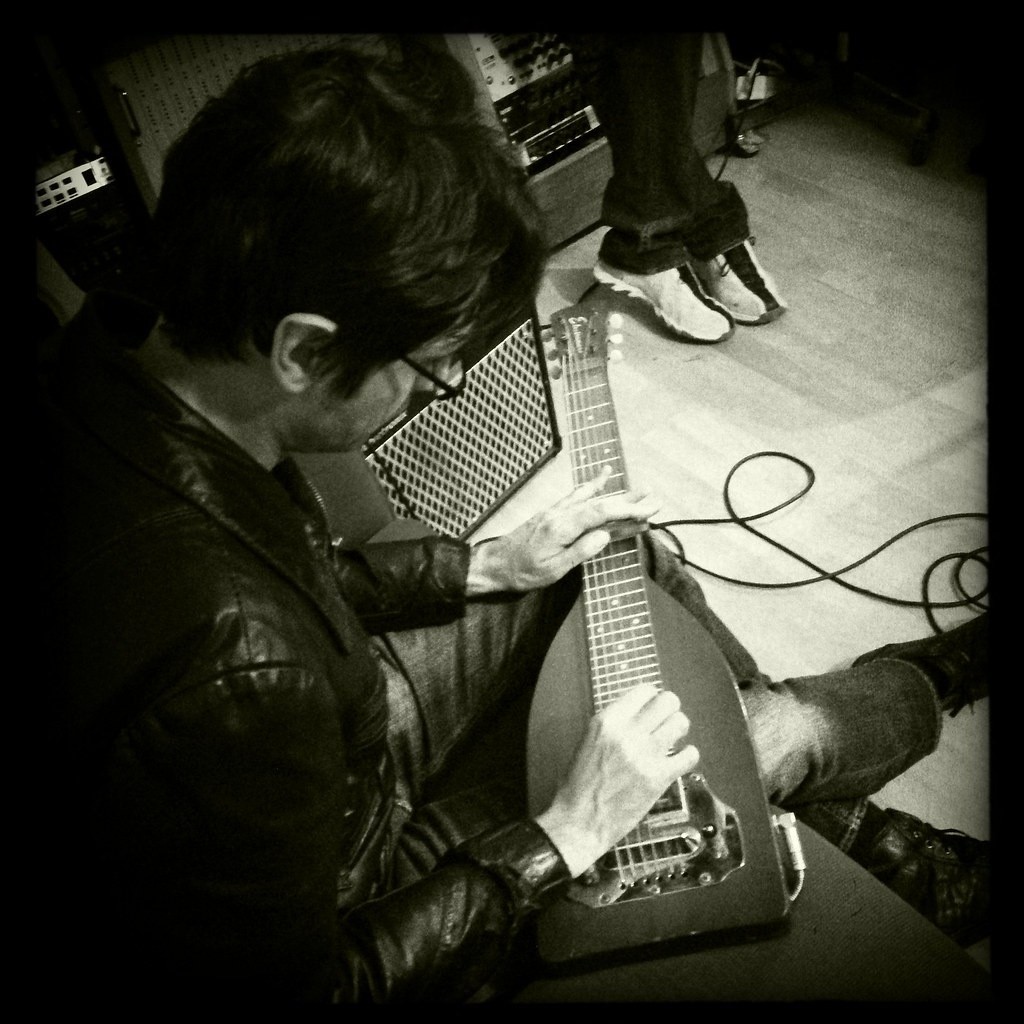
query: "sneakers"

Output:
[857,809,990,949]
[852,611,991,719]
[684,237,789,325]
[592,257,737,342]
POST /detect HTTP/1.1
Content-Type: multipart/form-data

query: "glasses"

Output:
[402,353,466,399]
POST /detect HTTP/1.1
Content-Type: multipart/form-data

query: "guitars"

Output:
[523,301,791,977]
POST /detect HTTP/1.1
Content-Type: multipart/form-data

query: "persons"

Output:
[0,38,995,1007]
[557,0,786,344]
